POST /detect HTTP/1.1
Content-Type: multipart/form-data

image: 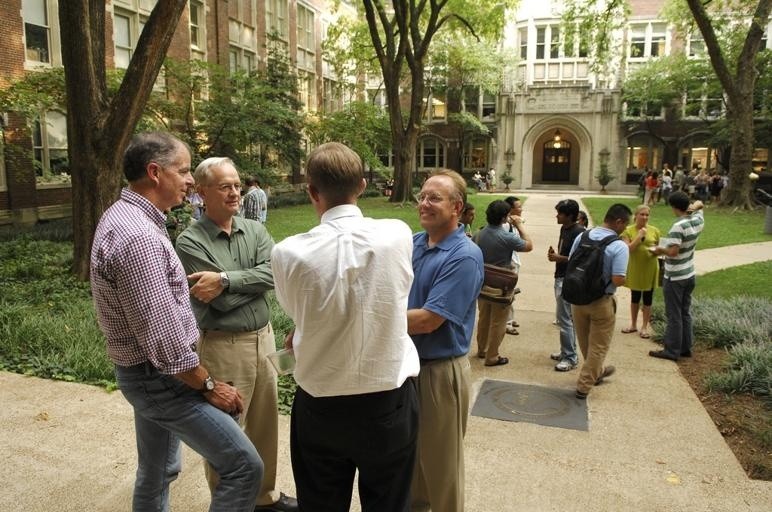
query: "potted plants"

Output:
[593,170,617,195]
[498,171,517,194]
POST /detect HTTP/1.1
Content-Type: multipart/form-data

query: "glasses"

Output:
[415,192,442,204]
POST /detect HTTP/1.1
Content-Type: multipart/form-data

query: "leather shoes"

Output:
[486,356,508,366]
[255,490,299,511]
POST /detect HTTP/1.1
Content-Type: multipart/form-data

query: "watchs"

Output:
[219,271,229,289]
[196,373,216,394]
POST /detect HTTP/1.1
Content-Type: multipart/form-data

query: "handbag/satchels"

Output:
[478,264,518,305]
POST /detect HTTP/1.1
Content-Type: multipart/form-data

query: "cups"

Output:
[266,348,293,377]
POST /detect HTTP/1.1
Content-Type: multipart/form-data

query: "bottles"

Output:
[549,245,554,253]
[508,217,526,223]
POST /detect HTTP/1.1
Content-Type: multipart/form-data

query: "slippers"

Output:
[622,328,637,333]
[640,332,649,338]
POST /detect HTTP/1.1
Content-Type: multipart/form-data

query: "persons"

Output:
[632,161,728,208]
[548,190,704,399]
[406,165,486,512]
[271,141,421,512]
[164,174,268,226]
[473,167,497,193]
[89,130,266,512]
[461,196,533,366]
[175,155,299,512]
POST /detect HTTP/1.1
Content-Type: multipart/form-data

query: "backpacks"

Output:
[562,229,622,305]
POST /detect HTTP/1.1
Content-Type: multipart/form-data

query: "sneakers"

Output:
[594,365,614,385]
[649,349,680,361]
[576,389,587,398]
[681,349,691,357]
[506,322,520,335]
[551,352,578,371]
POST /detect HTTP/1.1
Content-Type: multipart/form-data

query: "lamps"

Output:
[553,128,562,142]
